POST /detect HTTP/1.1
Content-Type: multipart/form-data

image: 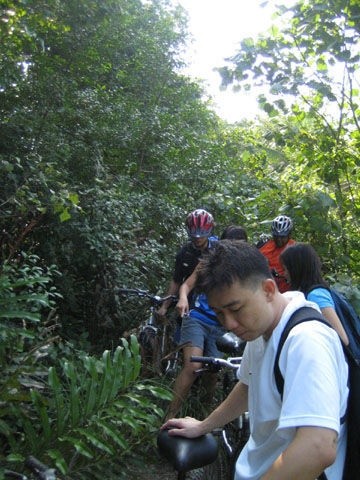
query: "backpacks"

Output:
[306,285,360,368]
[275,307,360,480]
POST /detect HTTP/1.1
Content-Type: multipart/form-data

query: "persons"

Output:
[279,241,360,366]
[164,225,248,421]
[154,208,218,409]
[160,239,360,480]
[259,215,295,294]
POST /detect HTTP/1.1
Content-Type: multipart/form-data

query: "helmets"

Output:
[185,209,213,238]
[272,216,293,236]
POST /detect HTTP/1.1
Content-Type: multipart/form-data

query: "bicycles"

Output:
[189,331,249,431]
[155,426,244,480]
[113,288,180,380]
